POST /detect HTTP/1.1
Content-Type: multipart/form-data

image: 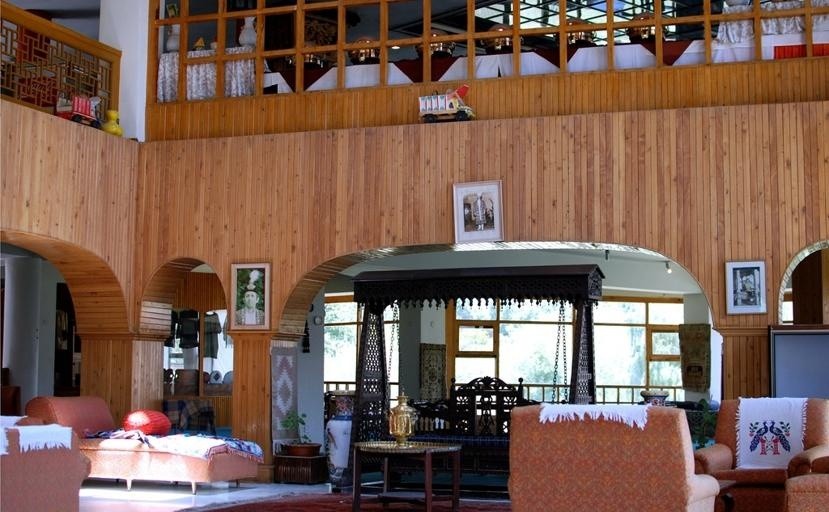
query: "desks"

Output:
[352,438,464,512]
[153,31,828,104]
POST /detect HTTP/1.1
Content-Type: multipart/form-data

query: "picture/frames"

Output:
[418,342,448,400]
[452,177,505,246]
[229,262,271,334]
[723,259,768,318]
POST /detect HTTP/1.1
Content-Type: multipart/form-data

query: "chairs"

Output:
[507,404,719,512]
[24,394,266,494]
[692,395,828,512]
[0,411,93,512]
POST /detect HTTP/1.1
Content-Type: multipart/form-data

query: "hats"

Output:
[244,284,259,294]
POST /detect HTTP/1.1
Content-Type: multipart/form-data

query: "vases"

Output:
[99,110,124,137]
[325,414,355,492]
[165,24,180,51]
[238,14,256,45]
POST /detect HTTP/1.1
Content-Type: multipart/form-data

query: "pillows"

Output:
[120,406,172,436]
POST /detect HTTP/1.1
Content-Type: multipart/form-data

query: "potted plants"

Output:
[280,408,322,456]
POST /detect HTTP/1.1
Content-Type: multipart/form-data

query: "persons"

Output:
[734,275,760,307]
[471,192,489,232]
[235,268,264,324]
